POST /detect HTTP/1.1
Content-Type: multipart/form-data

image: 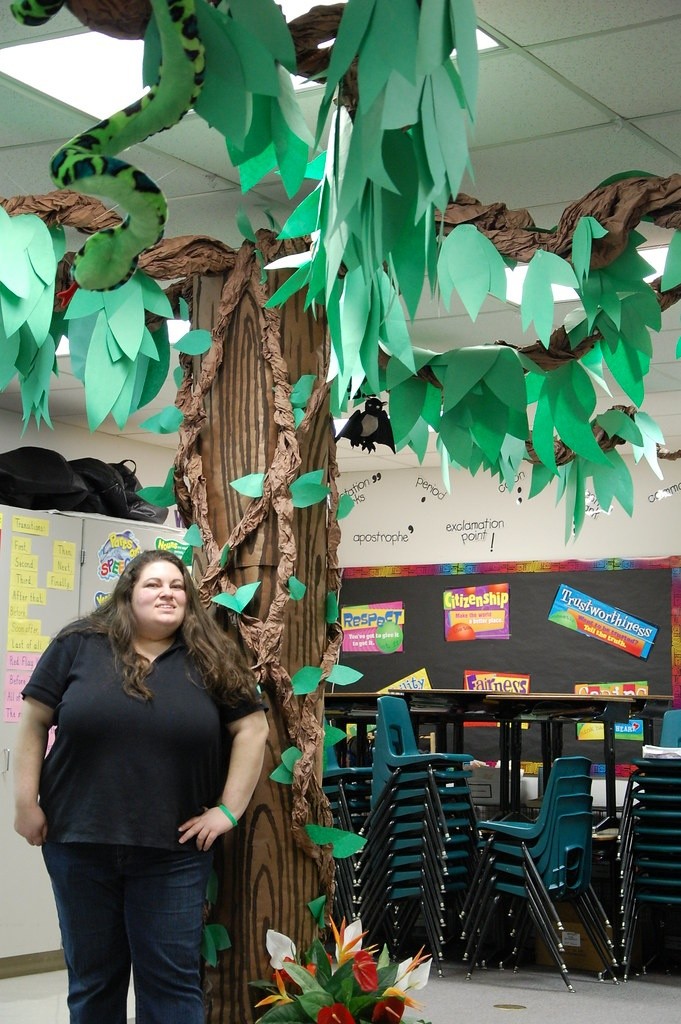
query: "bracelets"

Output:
[218,804,239,828]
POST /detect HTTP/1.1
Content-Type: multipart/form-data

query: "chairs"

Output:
[313,694,681,992]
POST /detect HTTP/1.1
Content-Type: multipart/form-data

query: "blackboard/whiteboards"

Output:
[320,556,681,780]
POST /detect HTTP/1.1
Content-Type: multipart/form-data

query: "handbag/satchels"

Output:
[0,446,169,525]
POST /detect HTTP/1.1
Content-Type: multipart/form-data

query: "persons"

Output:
[14,551,270,1024]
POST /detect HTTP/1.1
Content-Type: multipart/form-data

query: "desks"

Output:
[323,692,404,767]
[485,695,636,833]
[529,692,674,764]
[390,688,493,787]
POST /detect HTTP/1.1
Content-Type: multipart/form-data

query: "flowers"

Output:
[243,914,438,1024]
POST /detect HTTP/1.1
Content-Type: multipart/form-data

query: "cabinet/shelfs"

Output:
[0,502,195,982]
